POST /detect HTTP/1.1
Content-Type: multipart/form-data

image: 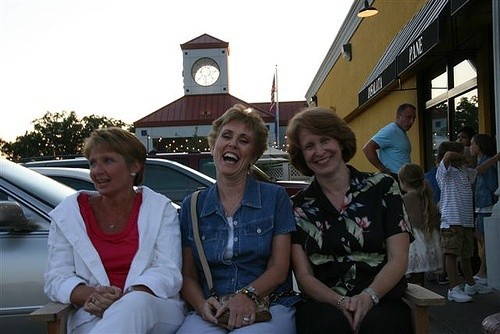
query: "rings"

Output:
[243,318,250,321]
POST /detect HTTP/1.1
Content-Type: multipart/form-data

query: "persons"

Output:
[43,127,187,334]
[283,107,415,334]
[177,104,301,334]
[363,104,500,304]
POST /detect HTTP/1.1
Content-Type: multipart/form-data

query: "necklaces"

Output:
[103,188,135,229]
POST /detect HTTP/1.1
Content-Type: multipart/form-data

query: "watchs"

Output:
[363,287,380,305]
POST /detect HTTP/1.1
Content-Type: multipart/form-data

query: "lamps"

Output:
[356,0,379,18]
[341,43,352,61]
[310,95,318,107]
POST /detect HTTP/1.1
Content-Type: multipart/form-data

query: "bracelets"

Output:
[237,287,259,305]
[337,296,345,309]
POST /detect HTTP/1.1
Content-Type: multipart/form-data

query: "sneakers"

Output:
[448,286,471,302]
[465,282,493,295]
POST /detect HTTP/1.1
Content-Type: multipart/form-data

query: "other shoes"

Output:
[439,272,448,285]
[473,274,487,285]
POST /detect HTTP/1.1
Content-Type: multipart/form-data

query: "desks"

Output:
[483,216,500,290]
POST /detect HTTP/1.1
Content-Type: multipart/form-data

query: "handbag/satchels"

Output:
[346,262,409,304]
[211,292,271,327]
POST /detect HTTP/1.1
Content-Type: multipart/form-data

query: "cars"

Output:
[0,152,181,334]
[17,152,311,207]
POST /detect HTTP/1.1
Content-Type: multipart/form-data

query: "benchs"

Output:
[28,283,445,334]
